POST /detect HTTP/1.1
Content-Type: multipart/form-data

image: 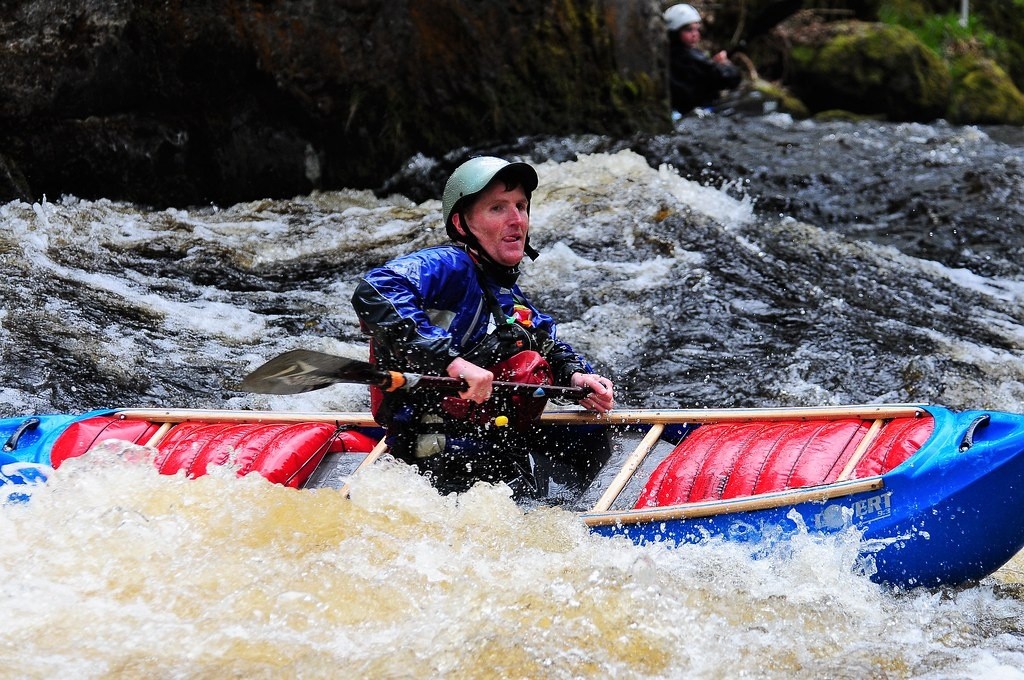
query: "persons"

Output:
[350,156,613,495]
[663,4,742,117]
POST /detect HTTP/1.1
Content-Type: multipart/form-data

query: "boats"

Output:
[716,53,806,117]
[0,407,1023,598]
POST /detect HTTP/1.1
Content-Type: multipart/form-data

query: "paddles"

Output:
[232,348,596,402]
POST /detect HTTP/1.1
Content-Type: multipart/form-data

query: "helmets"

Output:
[443,156,538,238]
[665,3,702,32]
